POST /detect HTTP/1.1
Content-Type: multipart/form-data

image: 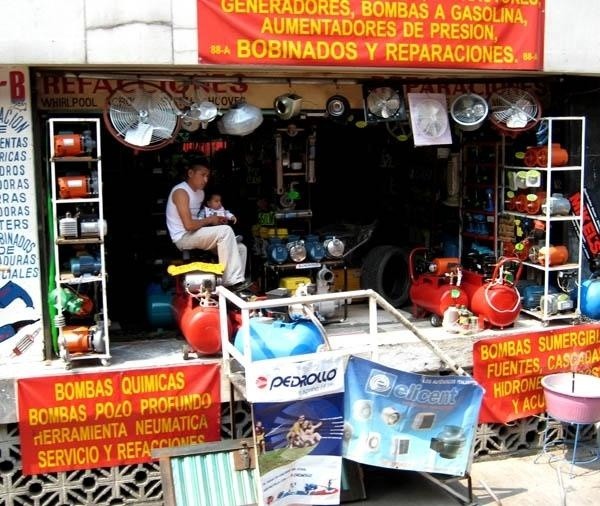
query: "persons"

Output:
[286,413,323,447]
[256,422,267,455]
[200,194,244,242]
[165,158,254,292]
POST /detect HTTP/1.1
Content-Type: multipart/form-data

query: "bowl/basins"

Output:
[540,371,600,425]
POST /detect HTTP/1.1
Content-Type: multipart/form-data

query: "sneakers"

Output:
[228,281,253,297]
[234,235,244,244]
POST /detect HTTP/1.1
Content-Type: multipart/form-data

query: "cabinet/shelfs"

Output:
[44,114,114,369]
[460,111,587,331]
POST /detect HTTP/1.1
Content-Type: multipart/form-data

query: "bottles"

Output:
[444,304,479,335]
